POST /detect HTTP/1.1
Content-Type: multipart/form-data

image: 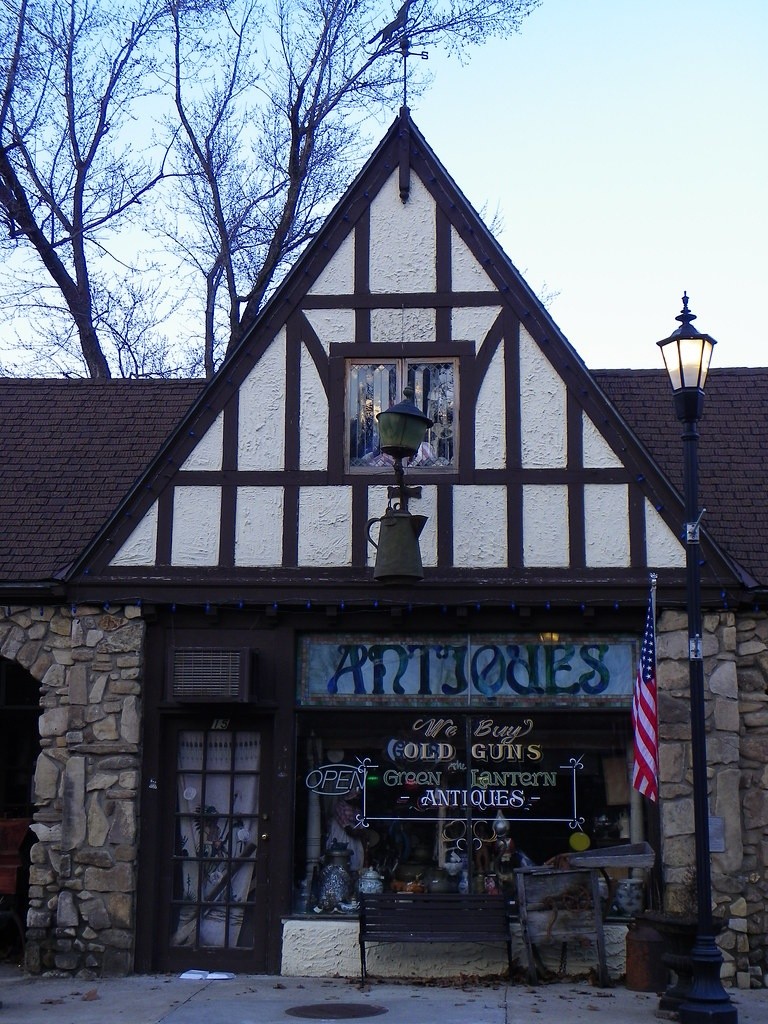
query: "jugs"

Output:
[364,503,428,580]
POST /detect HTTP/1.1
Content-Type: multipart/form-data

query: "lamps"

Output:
[538,632,560,643]
[376,386,435,475]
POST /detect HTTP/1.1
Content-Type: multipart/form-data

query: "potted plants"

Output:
[649,841,729,1011]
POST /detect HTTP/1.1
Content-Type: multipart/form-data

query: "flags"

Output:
[633,591,659,805]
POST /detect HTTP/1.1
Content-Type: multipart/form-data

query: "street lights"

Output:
[656,288,738,1024]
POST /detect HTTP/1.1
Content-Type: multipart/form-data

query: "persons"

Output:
[327,784,368,881]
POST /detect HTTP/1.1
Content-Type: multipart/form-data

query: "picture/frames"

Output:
[708,816,726,853]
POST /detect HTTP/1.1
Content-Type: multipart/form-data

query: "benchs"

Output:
[357,890,515,987]
